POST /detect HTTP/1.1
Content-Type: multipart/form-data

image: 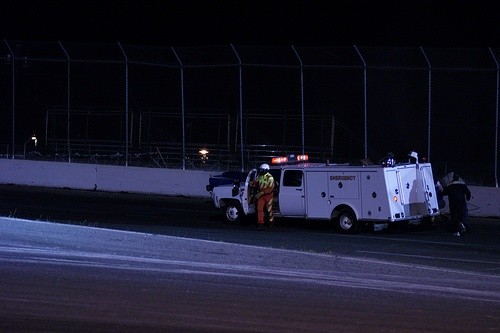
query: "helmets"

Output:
[260,164,270,171]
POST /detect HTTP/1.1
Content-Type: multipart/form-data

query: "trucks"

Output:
[206,155,441,235]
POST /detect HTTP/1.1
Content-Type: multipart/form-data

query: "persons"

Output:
[251,164,275,227]
[446,173,471,237]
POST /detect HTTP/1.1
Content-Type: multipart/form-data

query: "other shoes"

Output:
[256,225,263,230]
[457,232,461,236]
[269,225,273,231]
[461,224,466,229]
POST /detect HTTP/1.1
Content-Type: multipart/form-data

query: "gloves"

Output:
[254,193,261,199]
